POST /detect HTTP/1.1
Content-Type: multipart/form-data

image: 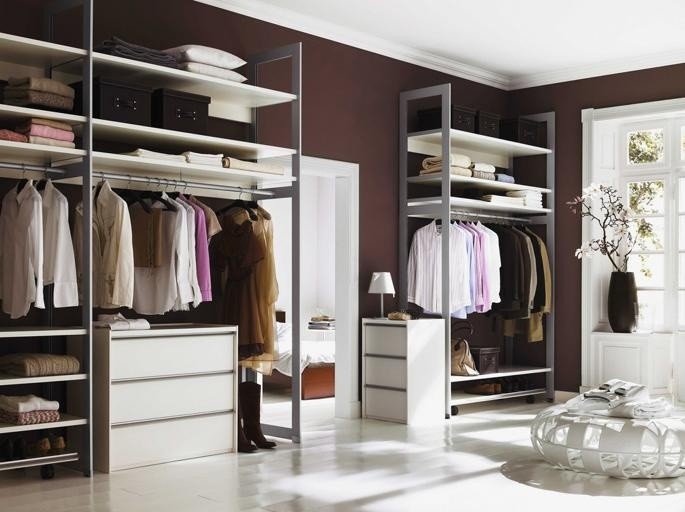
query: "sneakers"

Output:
[496,376,537,393]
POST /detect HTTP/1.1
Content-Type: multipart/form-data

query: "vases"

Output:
[606,269,641,333]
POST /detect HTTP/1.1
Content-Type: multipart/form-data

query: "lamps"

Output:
[367,270,397,319]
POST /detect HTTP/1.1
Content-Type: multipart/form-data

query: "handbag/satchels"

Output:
[451,318,481,378]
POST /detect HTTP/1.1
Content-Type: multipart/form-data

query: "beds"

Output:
[261,307,335,399]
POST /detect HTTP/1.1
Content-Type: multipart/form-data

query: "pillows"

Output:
[160,44,248,71]
[177,62,249,84]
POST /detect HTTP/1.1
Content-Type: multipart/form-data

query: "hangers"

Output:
[16,163,245,198]
[433,209,531,228]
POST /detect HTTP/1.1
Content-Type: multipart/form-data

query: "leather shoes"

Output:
[18,434,67,458]
[495,382,502,394]
[463,381,495,395]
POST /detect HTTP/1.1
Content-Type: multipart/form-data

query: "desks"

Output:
[529,403,685,481]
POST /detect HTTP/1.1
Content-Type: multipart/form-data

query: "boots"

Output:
[238,381,257,452]
[239,381,277,449]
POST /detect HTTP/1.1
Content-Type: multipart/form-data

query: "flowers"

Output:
[566,174,667,278]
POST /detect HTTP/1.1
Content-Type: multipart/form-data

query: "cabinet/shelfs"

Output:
[590,331,676,403]
[397,82,558,416]
[63,322,241,475]
[0,0,303,487]
[360,315,447,424]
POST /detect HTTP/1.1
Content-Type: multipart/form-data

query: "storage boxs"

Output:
[66,78,154,126]
[417,105,477,132]
[502,118,541,146]
[477,110,505,136]
[470,345,502,375]
[150,88,212,134]
